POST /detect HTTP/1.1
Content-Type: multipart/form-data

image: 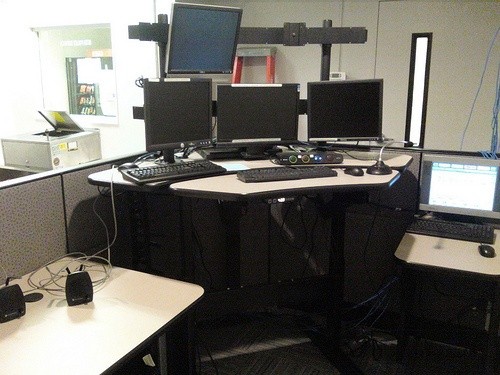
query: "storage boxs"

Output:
[2,111,102,171]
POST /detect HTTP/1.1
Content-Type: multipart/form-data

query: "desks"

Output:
[394,214,500,375]
[87,147,413,375]
[1,256,205,375]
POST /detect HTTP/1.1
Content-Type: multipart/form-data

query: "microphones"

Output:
[366,141,413,175]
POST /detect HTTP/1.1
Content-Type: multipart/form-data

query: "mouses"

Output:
[478,245,496,258]
[345,166,364,176]
[117,162,139,172]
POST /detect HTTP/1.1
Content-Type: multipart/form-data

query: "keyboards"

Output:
[237,165,337,183]
[120,160,226,185]
[404,218,494,244]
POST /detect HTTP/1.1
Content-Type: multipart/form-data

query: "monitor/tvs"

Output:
[164,3,243,79]
[418,151,500,223]
[216,83,300,161]
[143,78,213,166]
[307,78,384,152]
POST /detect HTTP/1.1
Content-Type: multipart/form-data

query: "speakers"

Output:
[0,277,26,323]
[64,265,93,306]
